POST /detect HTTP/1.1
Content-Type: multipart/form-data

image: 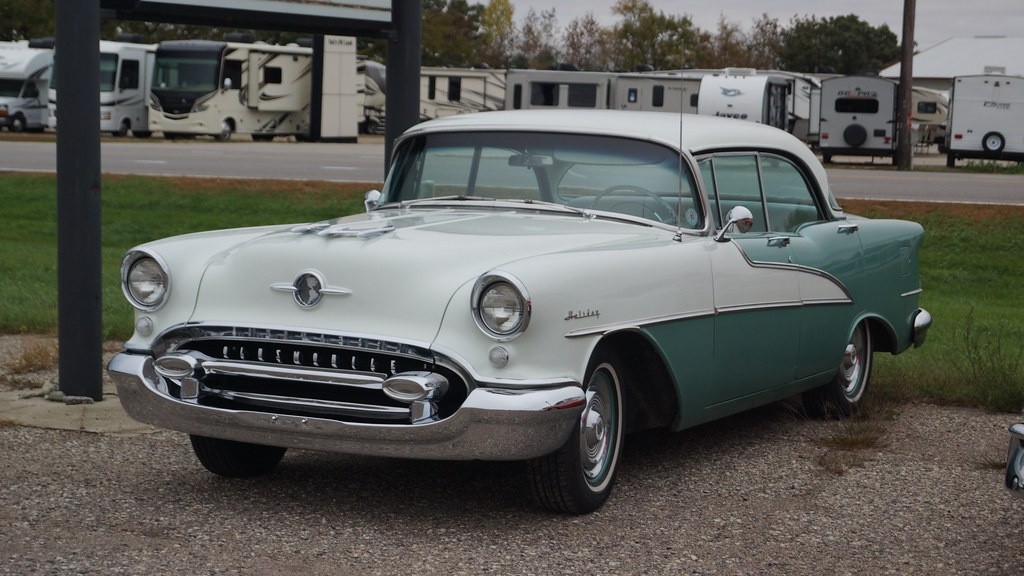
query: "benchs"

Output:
[566,196,817,233]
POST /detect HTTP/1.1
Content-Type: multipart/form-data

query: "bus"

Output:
[943,75,1024,168]
[1,34,360,142]
[418,64,899,168]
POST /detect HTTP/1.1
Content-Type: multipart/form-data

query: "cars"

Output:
[107,109,935,517]
